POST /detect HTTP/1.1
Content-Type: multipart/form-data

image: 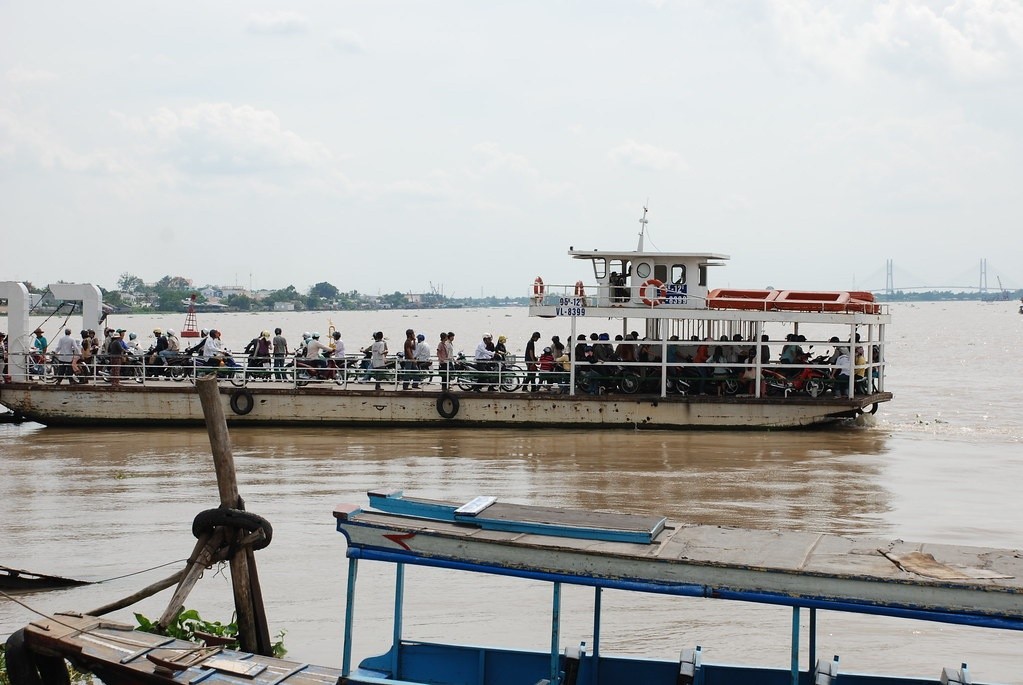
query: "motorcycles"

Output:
[759,356,829,398]
[244,346,273,381]
[360,346,434,385]
[284,348,358,386]
[450,348,526,392]
[674,362,740,395]
[578,356,641,394]
[98,343,147,383]
[146,342,249,386]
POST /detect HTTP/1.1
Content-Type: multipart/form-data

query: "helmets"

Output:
[416,334,425,340]
[857,346,864,354]
[839,346,848,354]
[599,334,608,339]
[543,347,551,352]
[615,331,769,342]
[790,334,800,342]
[483,333,492,340]
[830,337,840,342]
[499,335,507,340]
[80,327,415,339]
[799,335,807,341]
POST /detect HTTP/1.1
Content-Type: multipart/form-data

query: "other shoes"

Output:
[473,388,482,393]
[522,388,529,392]
[413,386,421,389]
[832,395,841,399]
[404,387,412,389]
[488,387,498,391]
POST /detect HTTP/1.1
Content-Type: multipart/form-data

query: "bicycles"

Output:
[38,357,91,384]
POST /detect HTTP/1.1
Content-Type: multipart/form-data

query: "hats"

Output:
[34,329,45,333]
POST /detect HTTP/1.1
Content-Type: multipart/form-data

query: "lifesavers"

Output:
[639,279,667,306]
[534,276,544,302]
[230,389,254,415]
[575,280,584,299]
[436,392,460,418]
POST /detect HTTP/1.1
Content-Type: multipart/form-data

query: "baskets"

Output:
[505,355,516,364]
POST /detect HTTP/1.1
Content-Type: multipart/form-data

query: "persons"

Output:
[575,331,656,394]
[360,331,395,385]
[188,329,227,381]
[610,273,624,302]
[245,331,273,382]
[273,328,288,382]
[474,333,508,391]
[694,334,770,396]
[522,332,576,393]
[780,333,807,376]
[102,327,144,386]
[667,336,681,388]
[437,332,455,390]
[148,329,181,380]
[828,332,866,399]
[299,331,345,378]
[403,329,430,390]
[34,329,47,375]
[0,332,8,381]
[52,329,99,384]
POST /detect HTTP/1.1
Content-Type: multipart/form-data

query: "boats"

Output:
[4,486,1023,685]
[0,198,895,428]
